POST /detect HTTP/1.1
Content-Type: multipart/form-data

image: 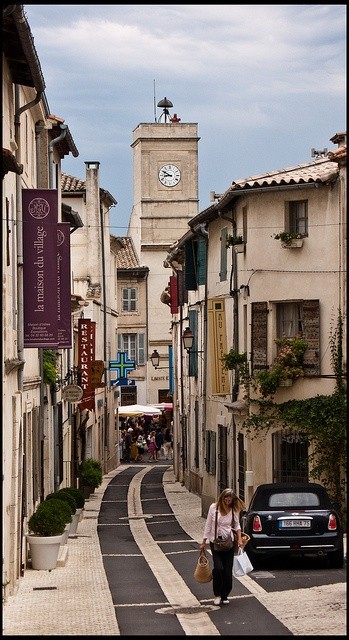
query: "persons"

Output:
[200,489,245,606]
[122,415,172,462]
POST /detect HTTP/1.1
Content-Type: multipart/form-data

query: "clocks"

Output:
[158,163,181,187]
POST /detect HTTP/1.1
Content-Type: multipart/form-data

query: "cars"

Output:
[244,482,343,569]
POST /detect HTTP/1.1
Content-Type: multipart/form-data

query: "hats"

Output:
[127,427,134,432]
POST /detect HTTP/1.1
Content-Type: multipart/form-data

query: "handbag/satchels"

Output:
[231,528,250,549]
[194,547,212,583]
[213,505,234,552]
[232,547,254,577]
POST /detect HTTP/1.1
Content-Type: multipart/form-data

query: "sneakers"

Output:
[223,599,230,604]
[147,460,153,463]
[213,597,221,605]
[155,461,158,462]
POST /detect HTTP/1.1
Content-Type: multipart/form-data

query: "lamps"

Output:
[181,328,195,354]
[151,349,160,370]
